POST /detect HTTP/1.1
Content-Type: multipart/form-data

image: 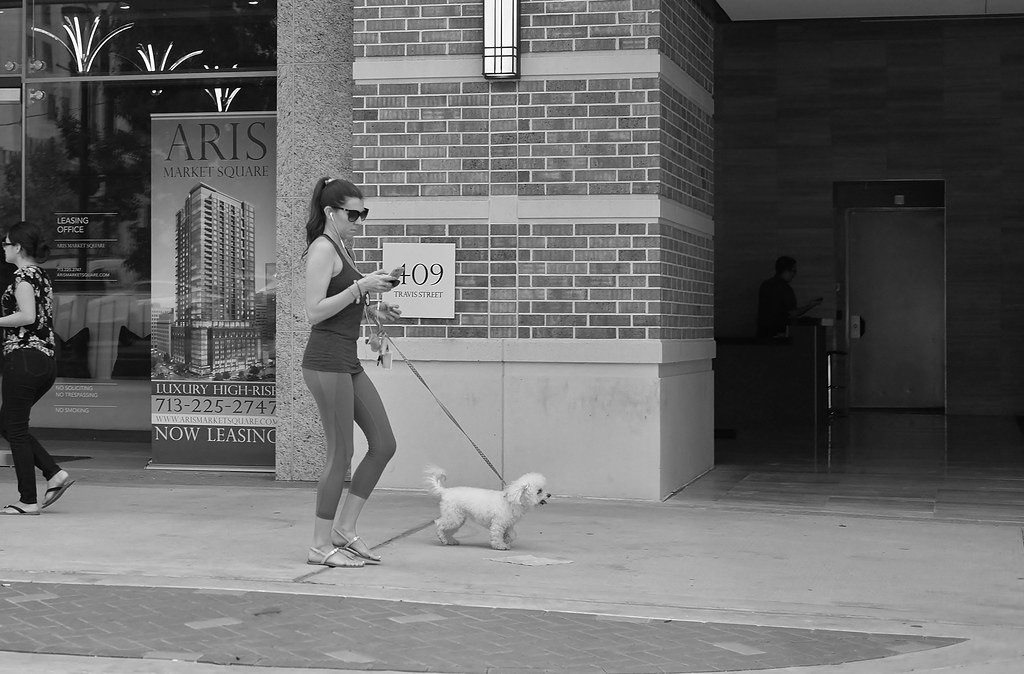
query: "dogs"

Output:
[419,462,553,552]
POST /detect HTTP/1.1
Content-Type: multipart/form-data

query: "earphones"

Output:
[330,213,334,222]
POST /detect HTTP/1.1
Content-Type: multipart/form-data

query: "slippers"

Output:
[41,479,76,508]
[1,505,41,516]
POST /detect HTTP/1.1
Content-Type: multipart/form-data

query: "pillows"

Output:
[110,324,151,381]
[54,326,93,379]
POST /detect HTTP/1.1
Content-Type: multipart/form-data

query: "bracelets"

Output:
[348,279,363,304]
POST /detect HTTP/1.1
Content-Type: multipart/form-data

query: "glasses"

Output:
[334,205,369,222]
[2,242,23,251]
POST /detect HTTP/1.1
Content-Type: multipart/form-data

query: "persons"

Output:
[0,222,76,516]
[301,177,404,567]
[754,256,823,340]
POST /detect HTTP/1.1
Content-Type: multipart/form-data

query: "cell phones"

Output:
[388,266,405,279]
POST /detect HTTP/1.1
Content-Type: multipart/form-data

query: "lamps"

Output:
[482,0,521,79]
[201,64,243,112]
[113,41,204,96]
[30,12,136,80]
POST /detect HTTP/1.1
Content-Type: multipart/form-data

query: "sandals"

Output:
[332,528,383,565]
[307,546,365,568]
